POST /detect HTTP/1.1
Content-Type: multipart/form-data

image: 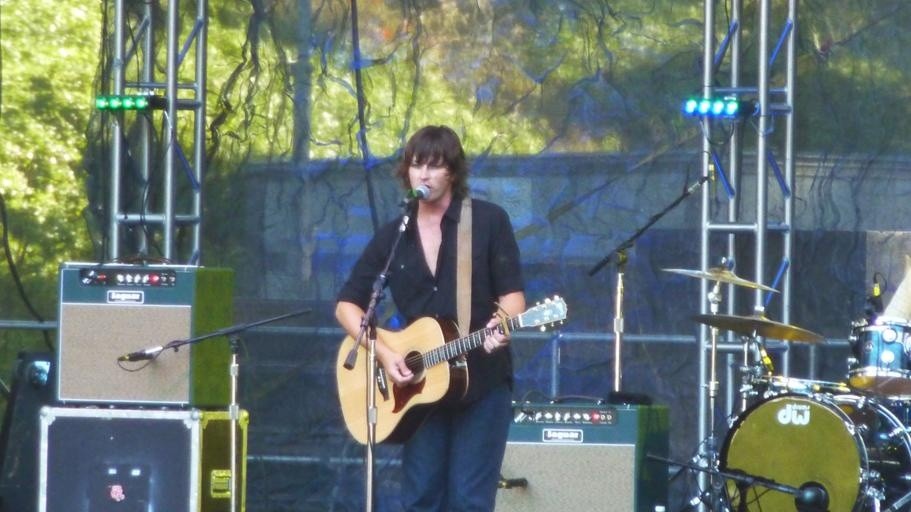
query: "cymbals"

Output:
[664,267,781,294]
[693,313,830,345]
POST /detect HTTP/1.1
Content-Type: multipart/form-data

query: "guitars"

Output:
[335,294,569,443]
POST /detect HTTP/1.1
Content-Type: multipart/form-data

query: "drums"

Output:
[764,374,852,401]
[874,396,911,433]
[712,391,911,512]
[847,319,911,396]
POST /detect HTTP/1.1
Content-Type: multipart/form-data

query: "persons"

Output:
[334,123,527,512]
[881,266,910,330]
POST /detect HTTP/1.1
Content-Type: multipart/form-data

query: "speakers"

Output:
[493,401,671,512]
[55,260,236,407]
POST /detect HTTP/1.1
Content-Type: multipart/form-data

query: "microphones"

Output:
[872,282,884,311]
[707,160,718,199]
[811,383,850,395]
[499,478,528,490]
[398,184,429,208]
[119,349,154,361]
[758,342,775,372]
[800,486,824,508]
